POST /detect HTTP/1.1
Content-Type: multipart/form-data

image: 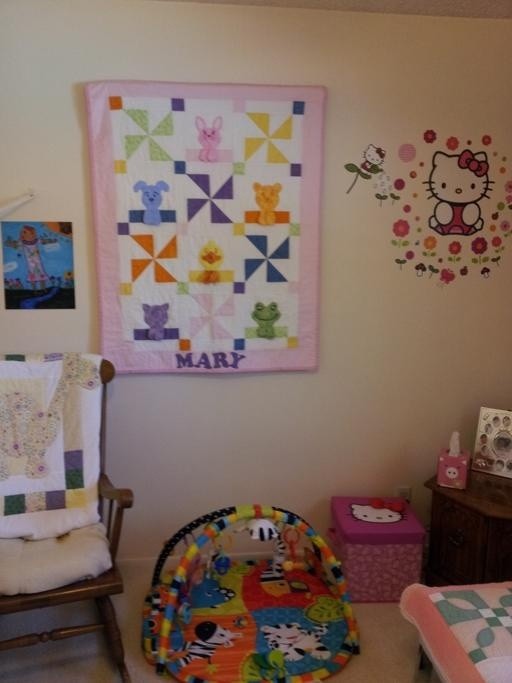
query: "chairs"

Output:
[0,353,133,683]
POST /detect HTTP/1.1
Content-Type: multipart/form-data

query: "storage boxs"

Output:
[327,497,427,603]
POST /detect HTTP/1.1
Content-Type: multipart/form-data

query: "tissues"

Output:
[435,430,472,491]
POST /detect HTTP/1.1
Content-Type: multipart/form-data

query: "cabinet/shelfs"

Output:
[426,491,512,588]
